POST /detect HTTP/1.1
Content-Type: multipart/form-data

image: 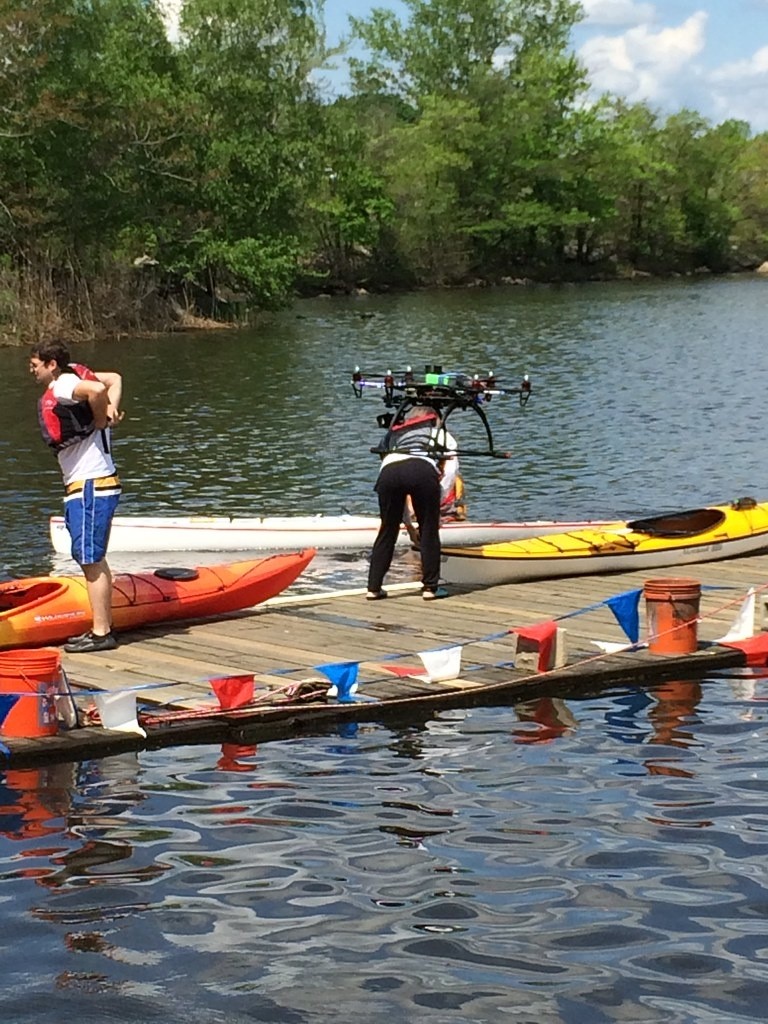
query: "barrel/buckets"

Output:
[643,578,703,656]
[0,649,59,739]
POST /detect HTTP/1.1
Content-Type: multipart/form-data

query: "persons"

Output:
[31,338,126,652]
[365,407,459,601]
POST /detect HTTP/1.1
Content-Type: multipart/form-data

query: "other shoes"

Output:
[422,588,448,599]
[365,590,388,600]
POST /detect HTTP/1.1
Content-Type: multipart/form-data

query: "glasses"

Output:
[29,360,44,371]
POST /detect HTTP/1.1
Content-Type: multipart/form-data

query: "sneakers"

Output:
[64,636,118,652]
[68,625,115,642]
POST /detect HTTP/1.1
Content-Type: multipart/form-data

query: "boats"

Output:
[3,552,316,650]
[47,514,637,557]
[440,500,766,580]
